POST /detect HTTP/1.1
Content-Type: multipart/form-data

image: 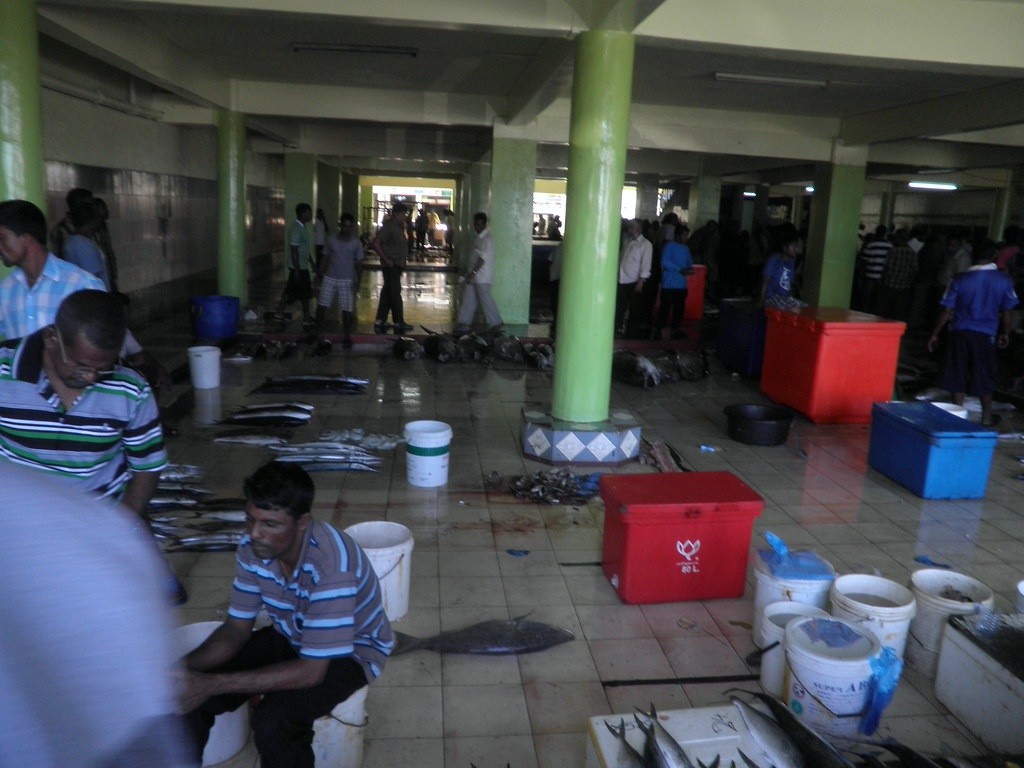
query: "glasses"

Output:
[54,324,121,383]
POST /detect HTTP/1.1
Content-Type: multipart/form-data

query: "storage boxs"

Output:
[584,704,785,767]
[932,615,1024,761]
[866,400,999,502]
[598,470,765,604]
[646,264,707,325]
[762,304,907,426]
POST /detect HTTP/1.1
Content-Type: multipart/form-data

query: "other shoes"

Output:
[374,319,394,329]
[272,314,292,323]
[393,323,414,332]
[489,323,506,333]
[344,335,353,347]
[653,329,663,341]
[615,328,625,338]
[670,330,689,341]
[302,316,317,325]
[453,327,474,334]
[305,331,318,345]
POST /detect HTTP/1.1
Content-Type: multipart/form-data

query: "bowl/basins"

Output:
[723,404,794,446]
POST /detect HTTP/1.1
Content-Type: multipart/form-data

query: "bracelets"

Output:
[473,271,477,275]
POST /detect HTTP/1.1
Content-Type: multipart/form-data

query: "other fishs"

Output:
[729,691,966,768]
[603,704,778,768]
[213,402,314,446]
[392,613,575,659]
[144,487,250,555]
[269,441,382,474]
[252,375,370,394]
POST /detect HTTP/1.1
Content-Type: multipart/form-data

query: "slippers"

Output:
[982,414,1002,427]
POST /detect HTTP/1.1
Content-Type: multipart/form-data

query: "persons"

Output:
[0,457,198,768]
[453,212,505,336]
[170,461,396,768]
[443,209,454,249]
[415,206,441,245]
[848,224,1024,428]
[373,203,414,329]
[0,289,170,518]
[614,212,811,379]
[533,213,562,240]
[547,245,560,330]
[382,210,391,223]
[316,208,329,265]
[0,200,107,342]
[300,212,364,350]
[272,202,321,325]
[52,188,118,292]
[406,208,414,242]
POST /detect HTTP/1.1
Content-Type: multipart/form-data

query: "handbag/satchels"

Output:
[118,328,142,358]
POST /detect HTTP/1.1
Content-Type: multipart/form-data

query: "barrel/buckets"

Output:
[310,685,369,768]
[1015,580,1024,613]
[405,482,450,538]
[170,622,251,767]
[187,346,222,389]
[403,419,453,489]
[341,520,414,622]
[190,294,239,339]
[750,546,994,751]
[192,387,224,428]
[932,402,967,418]
